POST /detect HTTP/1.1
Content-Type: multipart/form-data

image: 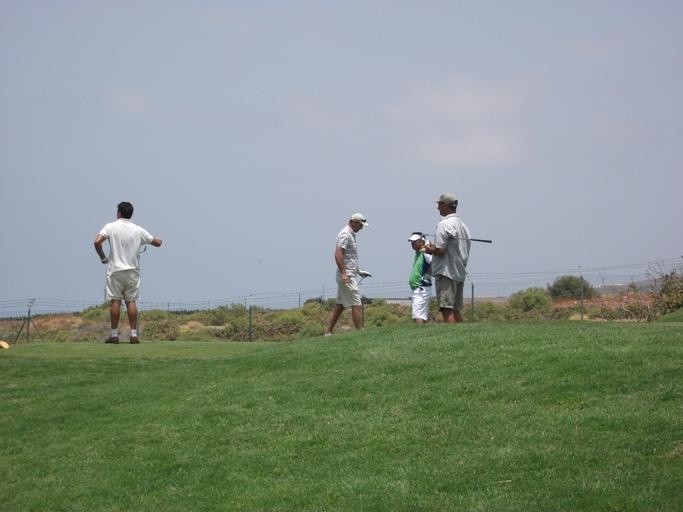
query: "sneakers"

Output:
[130,336,140,343]
[105,336,119,343]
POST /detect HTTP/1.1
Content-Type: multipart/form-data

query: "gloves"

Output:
[358,270,372,278]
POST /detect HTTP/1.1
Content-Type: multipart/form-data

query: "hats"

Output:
[433,193,458,207]
[408,234,425,242]
[350,214,369,226]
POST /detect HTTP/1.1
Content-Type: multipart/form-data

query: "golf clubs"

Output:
[357,273,371,287]
[419,233,492,244]
[104,245,146,260]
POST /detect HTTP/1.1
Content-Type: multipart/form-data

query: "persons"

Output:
[407,231,433,325]
[422,193,471,324]
[323,213,372,335]
[91,201,163,345]
